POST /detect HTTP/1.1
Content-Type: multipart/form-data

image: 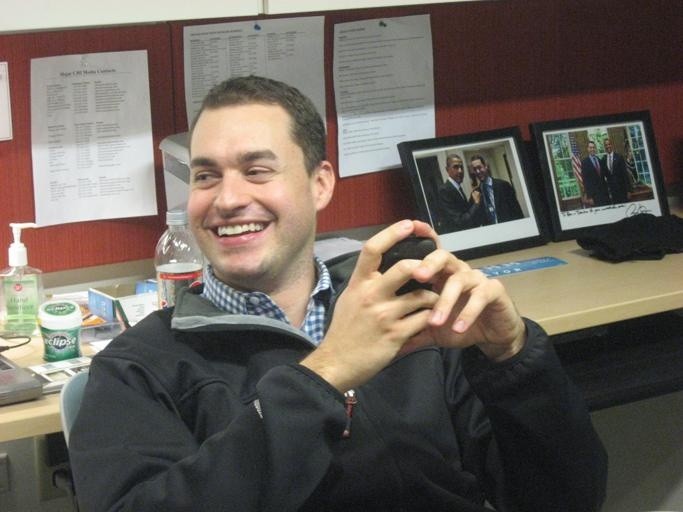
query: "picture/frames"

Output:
[528,107,671,244]
[396,123,554,262]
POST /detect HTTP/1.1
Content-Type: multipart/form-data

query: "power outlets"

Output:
[35,436,73,502]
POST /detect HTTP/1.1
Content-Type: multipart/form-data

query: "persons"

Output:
[435,155,481,234]
[621,140,640,187]
[598,138,630,205]
[467,155,524,229]
[581,140,611,209]
[68,72,611,512]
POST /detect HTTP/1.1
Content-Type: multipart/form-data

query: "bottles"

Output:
[155,211,204,313]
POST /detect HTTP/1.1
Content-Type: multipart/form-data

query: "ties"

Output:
[482,184,495,224]
[592,157,600,178]
[608,155,613,175]
[458,186,467,203]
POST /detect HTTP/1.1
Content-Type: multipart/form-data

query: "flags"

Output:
[569,132,584,195]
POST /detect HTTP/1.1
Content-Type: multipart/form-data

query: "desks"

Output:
[0,197,683,512]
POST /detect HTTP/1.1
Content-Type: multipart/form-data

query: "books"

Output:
[24,355,93,394]
[0,354,46,407]
[87,286,118,326]
[114,291,162,331]
